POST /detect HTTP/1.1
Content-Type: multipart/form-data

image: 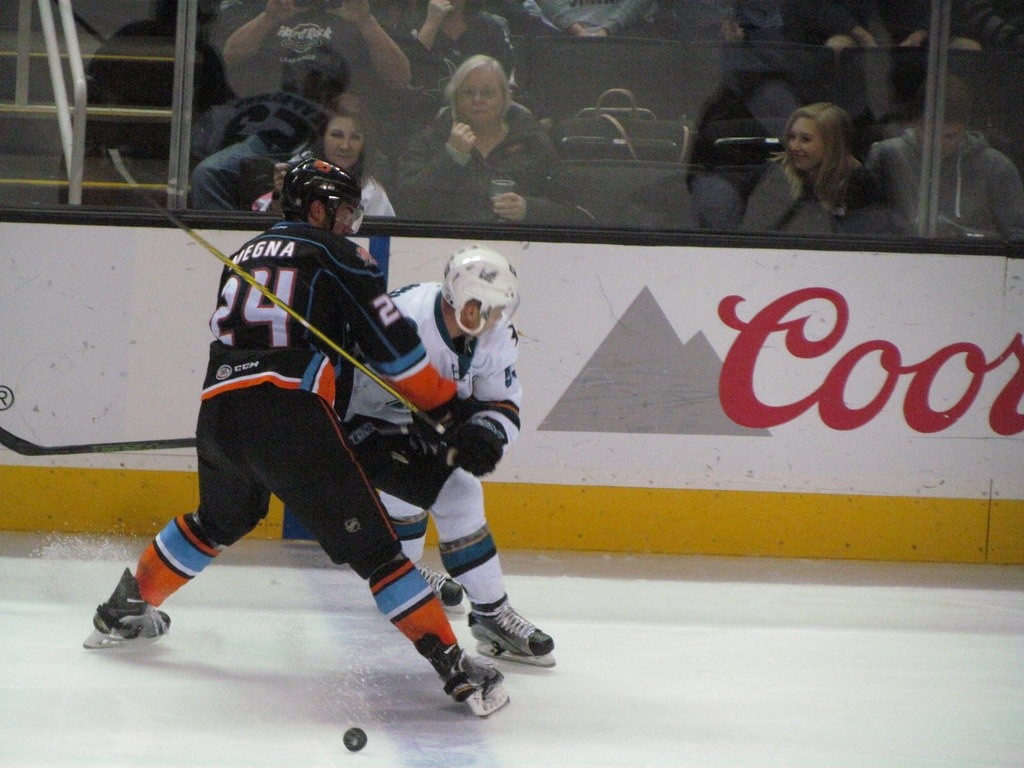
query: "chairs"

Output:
[0,0,1024,254]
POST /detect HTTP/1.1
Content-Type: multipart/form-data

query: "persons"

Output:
[81,158,554,716]
[57,0,1024,245]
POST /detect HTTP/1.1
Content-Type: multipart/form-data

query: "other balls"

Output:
[342,727,368,752]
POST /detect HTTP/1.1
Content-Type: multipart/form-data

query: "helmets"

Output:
[283,157,361,219]
[441,245,519,336]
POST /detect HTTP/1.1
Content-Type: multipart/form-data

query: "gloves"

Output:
[456,413,515,477]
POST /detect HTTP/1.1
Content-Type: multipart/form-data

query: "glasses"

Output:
[457,85,507,99]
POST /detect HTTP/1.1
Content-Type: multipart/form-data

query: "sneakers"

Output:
[410,557,464,617]
[415,632,510,717]
[81,568,171,652]
[471,592,557,665]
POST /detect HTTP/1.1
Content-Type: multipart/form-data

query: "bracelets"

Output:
[264,7,283,28]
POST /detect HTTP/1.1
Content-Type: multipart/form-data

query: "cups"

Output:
[492,179,515,205]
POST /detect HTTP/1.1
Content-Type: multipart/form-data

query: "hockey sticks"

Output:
[1,425,430,456]
[109,146,459,442]
